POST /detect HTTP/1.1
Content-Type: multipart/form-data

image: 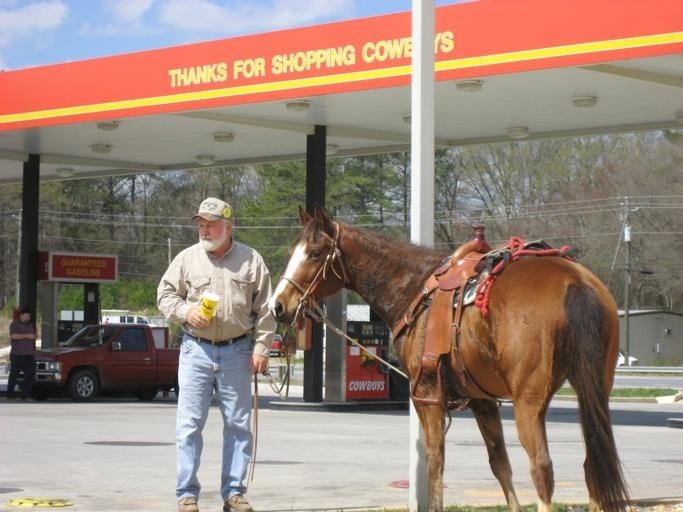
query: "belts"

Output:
[184,331,246,346]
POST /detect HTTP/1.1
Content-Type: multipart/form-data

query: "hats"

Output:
[191,198,233,224]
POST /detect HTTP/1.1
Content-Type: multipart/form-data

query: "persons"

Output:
[156,197,279,512]
[7,308,37,403]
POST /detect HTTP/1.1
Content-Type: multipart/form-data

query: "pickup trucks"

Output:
[15,323,180,403]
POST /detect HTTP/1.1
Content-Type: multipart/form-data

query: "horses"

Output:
[267,201,634,512]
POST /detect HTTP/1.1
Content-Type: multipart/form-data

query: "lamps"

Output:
[53,168,75,179]
[570,95,599,110]
[284,100,311,113]
[326,144,340,156]
[89,120,119,155]
[195,132,234,166]
[401,81,532,141]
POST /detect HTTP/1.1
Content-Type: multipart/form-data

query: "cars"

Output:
[270,334,286,357]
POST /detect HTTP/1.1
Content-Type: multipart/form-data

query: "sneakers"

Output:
[6,396,36,403]
[178,496,199,512]
[223,494,252,512]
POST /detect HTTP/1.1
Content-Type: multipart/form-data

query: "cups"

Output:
[199,292,220,325]
